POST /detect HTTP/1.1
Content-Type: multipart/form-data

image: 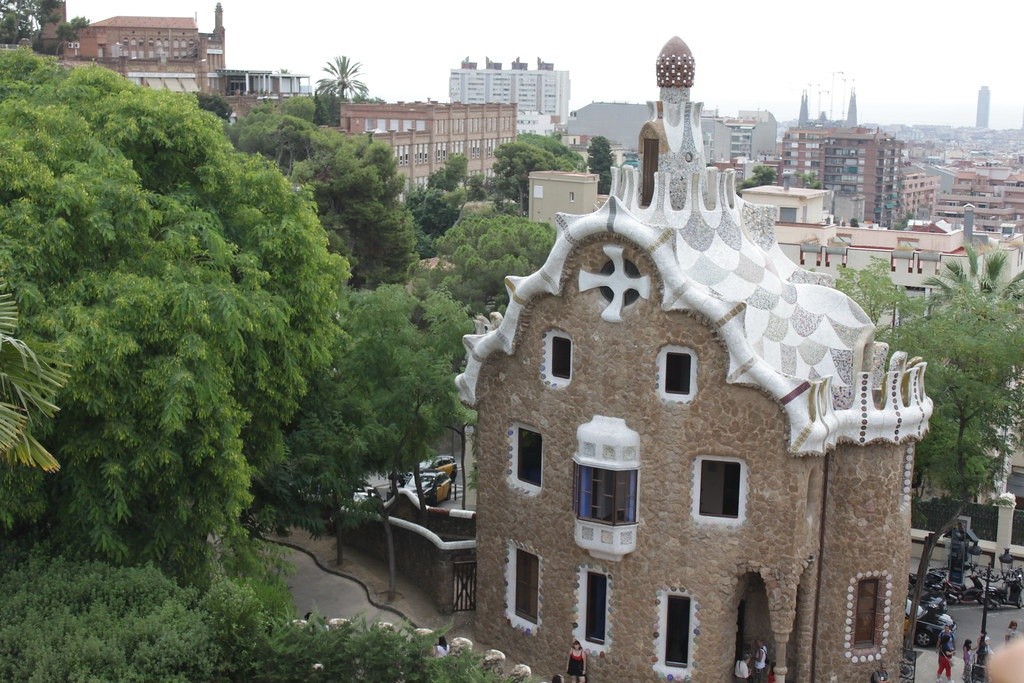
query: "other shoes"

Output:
[947,679,954,683]
[936,678,945,683]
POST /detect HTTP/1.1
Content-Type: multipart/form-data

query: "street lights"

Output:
[968,540,1013,665]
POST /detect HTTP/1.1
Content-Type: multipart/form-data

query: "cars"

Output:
[902,598,957,648]
[420,455,457,483]
[404,470,452,506]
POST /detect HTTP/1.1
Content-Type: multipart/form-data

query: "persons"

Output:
[871,661,892,683]
[936,625,957,683]
[984,633,1024,683]
[566,639,587,683]
[304,611,327,631]
[963,638,979,683]
[975,629,994,683]
[904,612,910,642]
[735,639,776,683]
[437,635,450,657]
[1005,620,1018,644]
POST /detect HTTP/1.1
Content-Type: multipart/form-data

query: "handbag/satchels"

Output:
[765,655,769,664]
[949,656,956,666]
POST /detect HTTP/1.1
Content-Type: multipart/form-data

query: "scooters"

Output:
[909,561,1024,610]
